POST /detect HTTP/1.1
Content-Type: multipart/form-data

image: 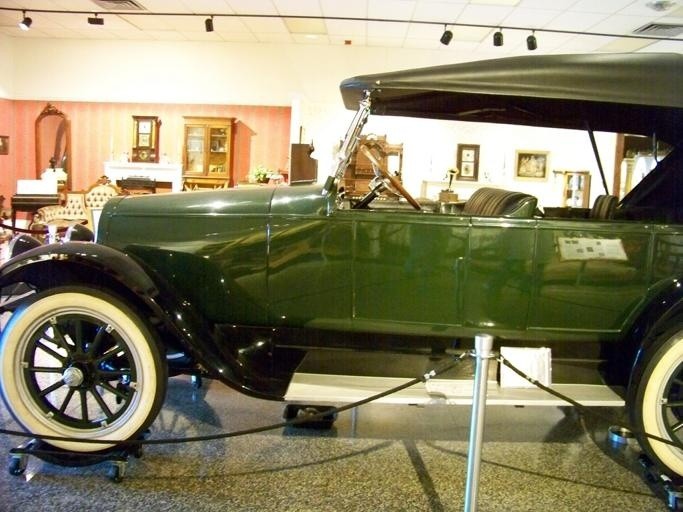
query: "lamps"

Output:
[19,11,32,31]
[88,14,104,25]
[494,28,503,46]
[205,16,214,32]
[527,30,537,50]
[440,24,453,45]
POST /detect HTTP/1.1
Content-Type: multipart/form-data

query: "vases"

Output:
[256,175,270,184]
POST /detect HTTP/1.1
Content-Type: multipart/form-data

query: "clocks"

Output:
[132,115,161,163]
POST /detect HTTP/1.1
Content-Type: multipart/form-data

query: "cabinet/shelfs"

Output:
[180,116,236,191]
[338,133,403,201]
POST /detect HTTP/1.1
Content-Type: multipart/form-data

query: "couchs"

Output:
[28,174,152,244]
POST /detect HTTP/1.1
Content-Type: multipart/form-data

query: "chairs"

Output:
[462,188,537,216]
[590,194,619,220]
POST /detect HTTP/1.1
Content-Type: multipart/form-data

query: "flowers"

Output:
[254,165,273,181]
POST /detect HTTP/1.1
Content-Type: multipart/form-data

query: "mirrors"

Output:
[35,101,71,191]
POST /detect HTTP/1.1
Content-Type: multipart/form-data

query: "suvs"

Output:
[0,44,679,489]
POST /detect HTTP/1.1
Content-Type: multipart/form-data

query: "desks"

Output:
[0,219,88,245]
[10,196,60,230]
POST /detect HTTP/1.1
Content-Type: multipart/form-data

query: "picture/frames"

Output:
[0,136,9,155]
[456,144,480,181]
[513,150,549,182]
